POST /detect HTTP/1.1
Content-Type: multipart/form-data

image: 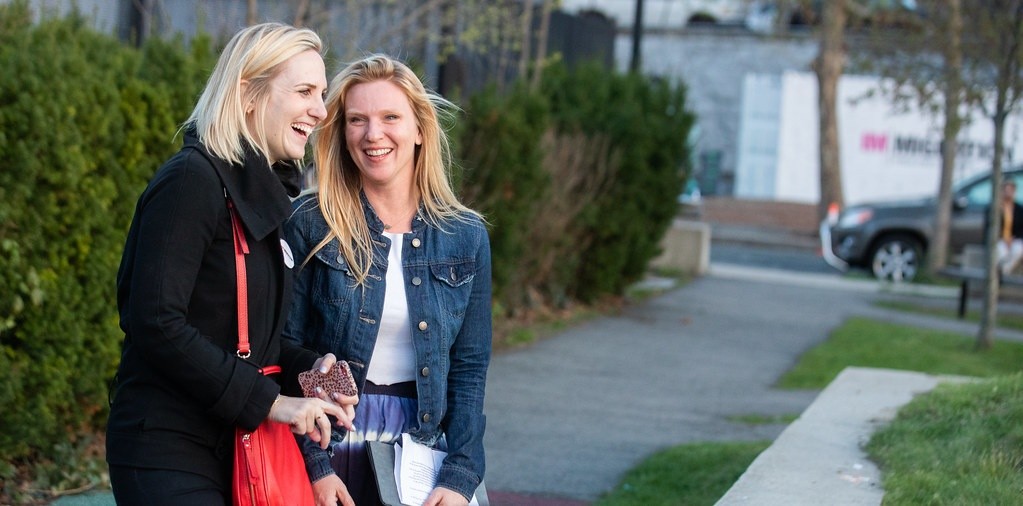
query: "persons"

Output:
[984,180,1023,286]
[281,51,492,506]
[106,23,359,506]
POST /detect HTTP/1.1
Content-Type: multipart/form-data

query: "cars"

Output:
[830,166,1023,283]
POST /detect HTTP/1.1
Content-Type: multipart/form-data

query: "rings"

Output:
[315,413,325,421]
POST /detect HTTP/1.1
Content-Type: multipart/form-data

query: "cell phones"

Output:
[298,360,358,403]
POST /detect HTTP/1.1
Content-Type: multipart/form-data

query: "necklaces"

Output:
[384,205,416,230]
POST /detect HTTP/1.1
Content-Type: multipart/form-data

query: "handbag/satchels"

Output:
[234,419,316,506]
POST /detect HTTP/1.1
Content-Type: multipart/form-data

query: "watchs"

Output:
[273,393,280,405]
